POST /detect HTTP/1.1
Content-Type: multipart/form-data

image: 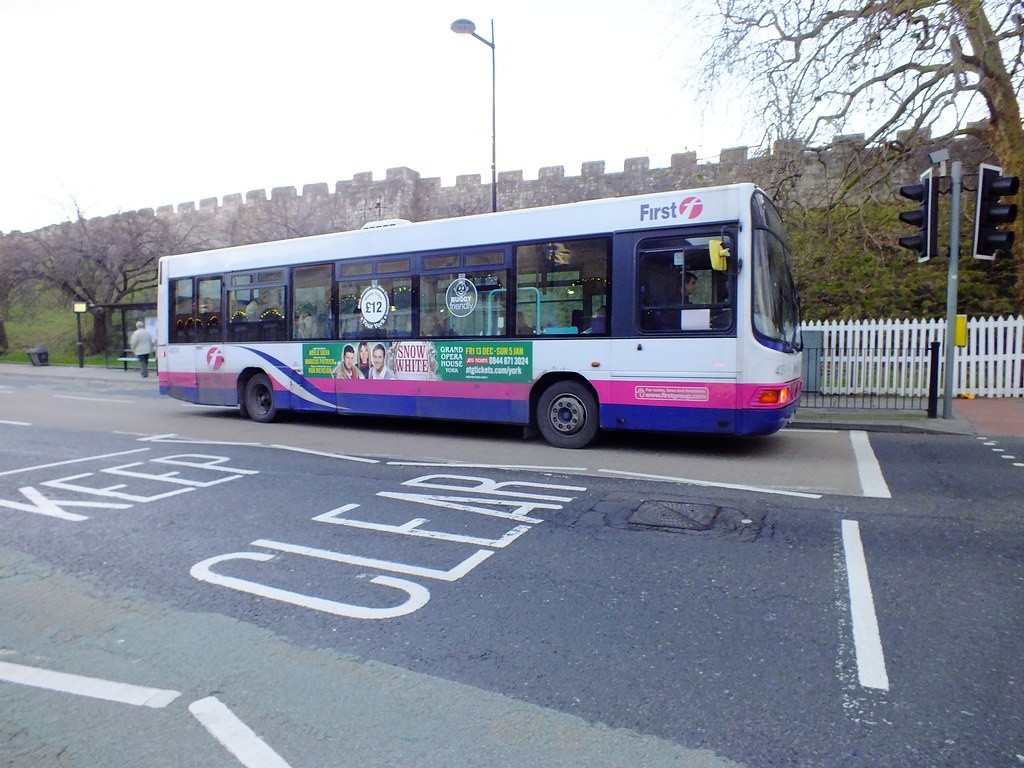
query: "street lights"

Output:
[452,18,498,214]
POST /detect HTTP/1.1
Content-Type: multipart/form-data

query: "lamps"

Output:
[72,301,86,312]
[451,19,475,34]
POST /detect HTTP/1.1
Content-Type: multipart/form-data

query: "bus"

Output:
[153,182,807,449]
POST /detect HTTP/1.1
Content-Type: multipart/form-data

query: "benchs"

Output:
[117,349,156,362]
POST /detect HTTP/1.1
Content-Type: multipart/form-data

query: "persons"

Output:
[500,312,534,335]
[672,274,699,327]
[333,342,396,380]
[420,308,450,336]
[130,319,155,378]
[245,299,334,340]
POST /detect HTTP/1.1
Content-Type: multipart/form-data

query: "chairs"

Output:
[179,308,542,336]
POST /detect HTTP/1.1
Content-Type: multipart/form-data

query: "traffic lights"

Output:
[899,167,933,264]
[971,163,1020,262]
